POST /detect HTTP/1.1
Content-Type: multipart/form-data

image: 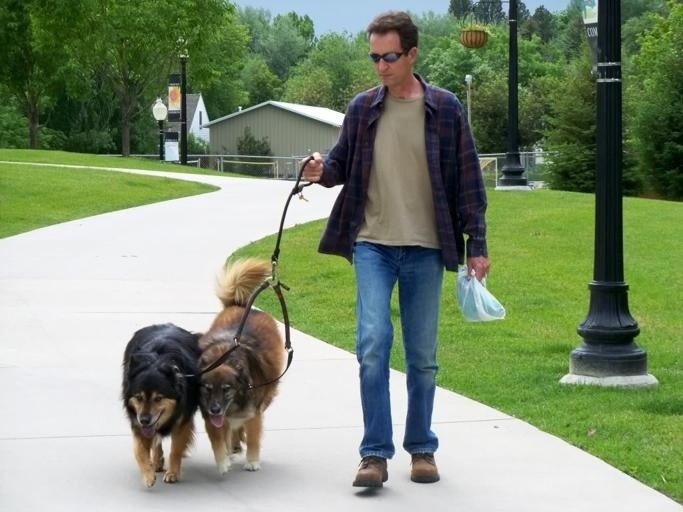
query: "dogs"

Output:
[197,252,286,474]
[119,322,204,489]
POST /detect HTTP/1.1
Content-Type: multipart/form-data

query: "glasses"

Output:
[368,52,404,62]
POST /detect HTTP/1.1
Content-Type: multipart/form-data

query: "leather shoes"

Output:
[411,453,440,483]
[353,456,388,487]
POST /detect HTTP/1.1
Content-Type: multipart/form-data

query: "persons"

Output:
[300,10,490,487]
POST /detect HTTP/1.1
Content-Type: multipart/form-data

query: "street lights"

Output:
[174,36,189,166]
[152,97,168,160]
